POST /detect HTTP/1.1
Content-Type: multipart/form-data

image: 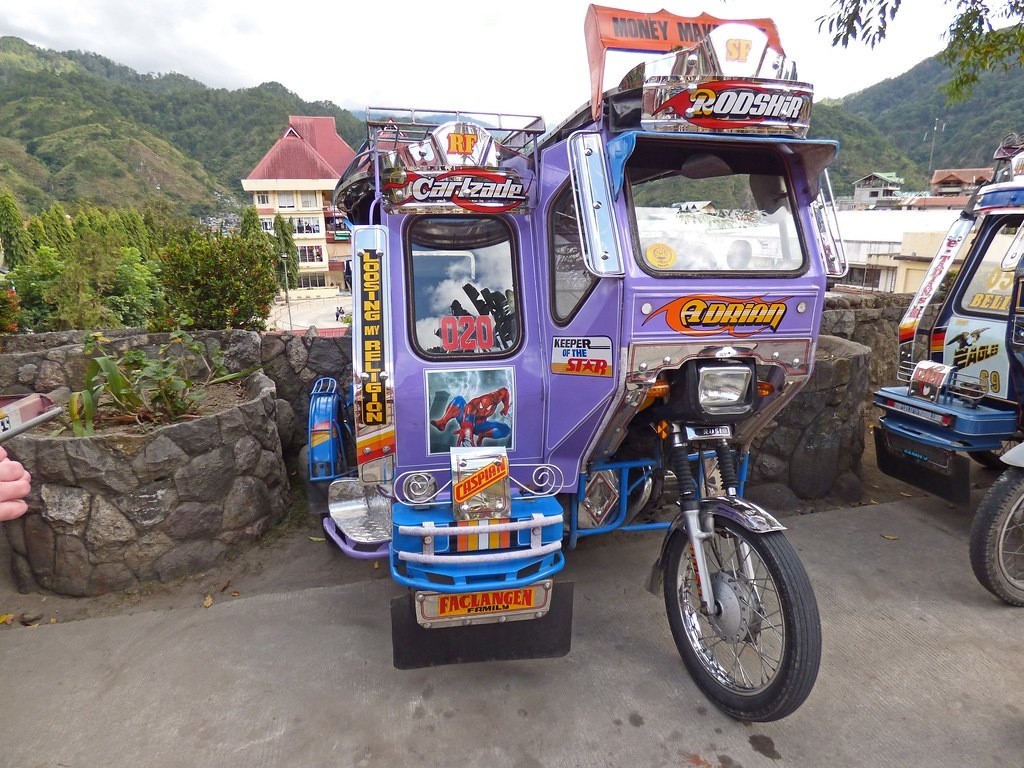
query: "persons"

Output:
[335,306,346,322]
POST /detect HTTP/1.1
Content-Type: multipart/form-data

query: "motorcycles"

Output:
[310,1,848,727]
[869,155,1024,616]
